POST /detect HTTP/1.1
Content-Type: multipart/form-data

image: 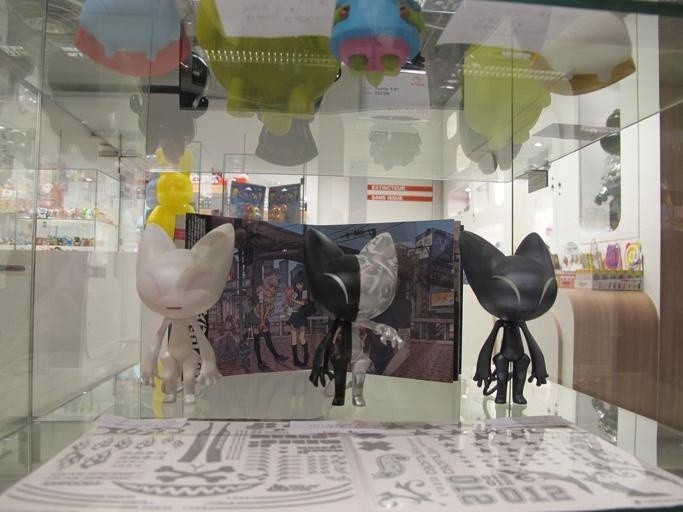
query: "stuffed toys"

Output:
[75,1,636,171]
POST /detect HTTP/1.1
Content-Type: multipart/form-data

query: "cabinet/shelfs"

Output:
[0,0,683,512]
[12,170,120,252]
[461,284,660,420]
